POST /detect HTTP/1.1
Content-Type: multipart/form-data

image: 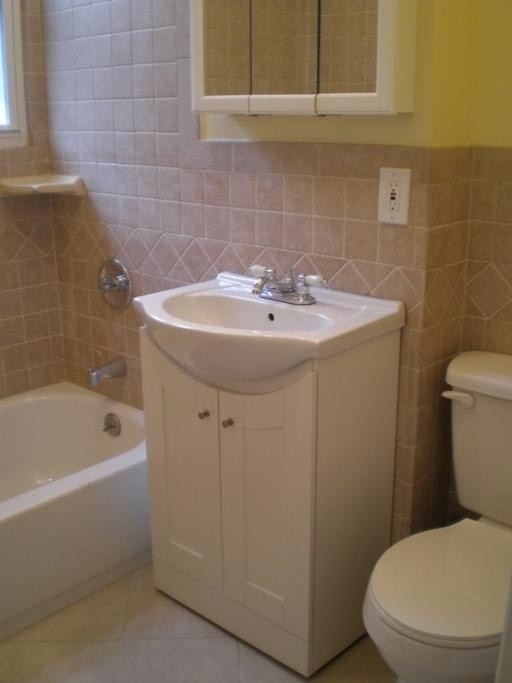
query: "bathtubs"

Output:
[0,381,151,645]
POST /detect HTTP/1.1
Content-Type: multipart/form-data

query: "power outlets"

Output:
[377,167,412,226]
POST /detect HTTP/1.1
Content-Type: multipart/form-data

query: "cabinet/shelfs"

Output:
[137,327,402,678]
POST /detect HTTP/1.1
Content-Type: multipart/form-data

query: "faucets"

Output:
[251,268,295,300]
[88,357,127,386]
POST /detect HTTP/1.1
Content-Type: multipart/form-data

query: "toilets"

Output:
[362,352,511,683]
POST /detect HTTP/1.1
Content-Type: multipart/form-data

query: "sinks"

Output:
[132,270,405,381]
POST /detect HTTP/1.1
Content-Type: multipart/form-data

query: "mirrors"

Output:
[189,0,418,117]
